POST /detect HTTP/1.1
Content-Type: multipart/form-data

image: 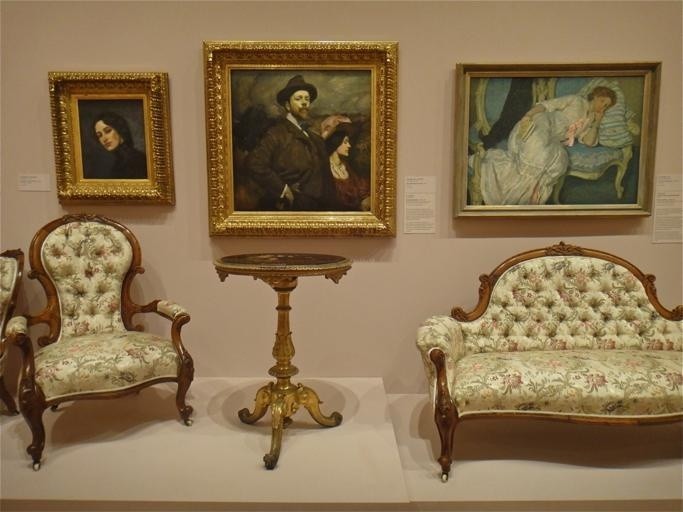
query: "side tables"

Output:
[211,255,357,471]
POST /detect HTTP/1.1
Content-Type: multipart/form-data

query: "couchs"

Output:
[412,245,683,484]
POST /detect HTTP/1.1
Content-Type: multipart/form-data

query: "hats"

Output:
[276,74,318,106]
[320,114,353,142]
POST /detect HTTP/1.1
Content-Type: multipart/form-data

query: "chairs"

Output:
[1,247,27,416]
[7,212,204,474]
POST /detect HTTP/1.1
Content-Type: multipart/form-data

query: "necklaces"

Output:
[327,159,346,178]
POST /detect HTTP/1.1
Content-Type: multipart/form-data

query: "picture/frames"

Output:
[452,58,662,222]
[201,39,399,240]
[46,71,175,210]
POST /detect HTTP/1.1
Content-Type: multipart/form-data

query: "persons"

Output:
[243,75,339,211]
[470,87,617,206]
[324,130,369,211]
[91,111,147,179]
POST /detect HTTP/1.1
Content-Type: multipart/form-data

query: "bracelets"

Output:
[523,114,531,118]
